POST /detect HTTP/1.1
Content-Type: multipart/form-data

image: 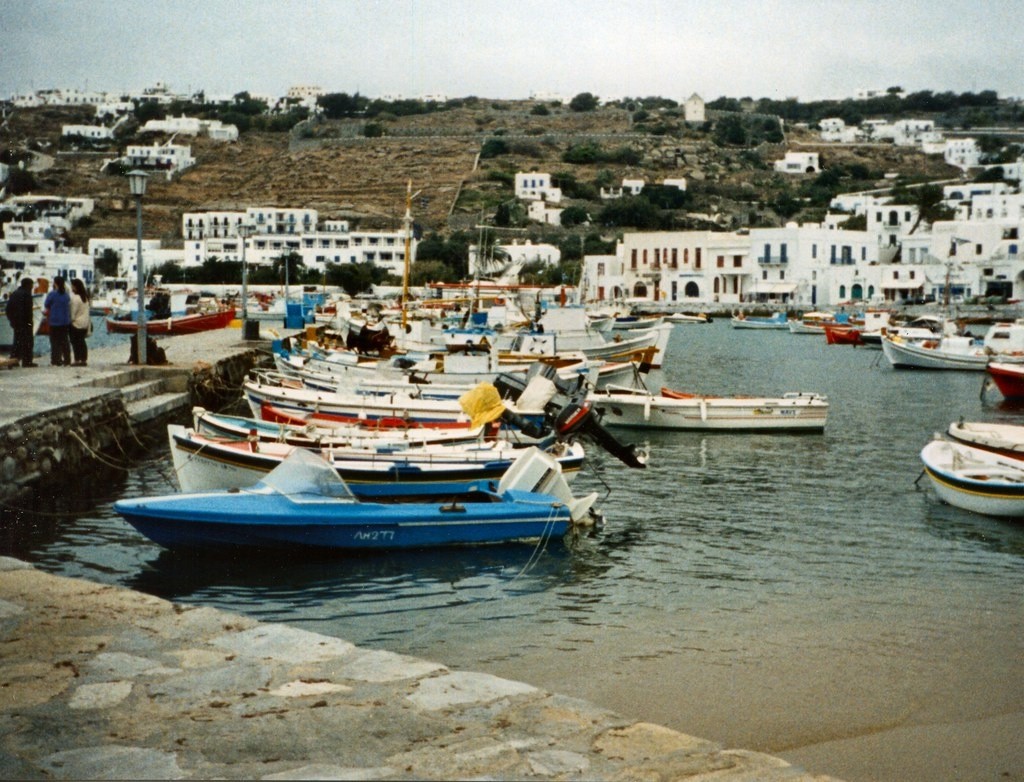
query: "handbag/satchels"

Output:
[83,320,93,337]
[37,314,50,335]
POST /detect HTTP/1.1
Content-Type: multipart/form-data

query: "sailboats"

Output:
[859,238,984,349]
[299,178,584,368]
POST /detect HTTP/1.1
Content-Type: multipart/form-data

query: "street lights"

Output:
[282,246,293,302]
[237,223,250,320]
[126,169,152,363]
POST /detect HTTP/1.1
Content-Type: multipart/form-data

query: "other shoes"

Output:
[71,362,87,366]
[22,361,37,368]
[62,360,70,365]
[50,360,62,365]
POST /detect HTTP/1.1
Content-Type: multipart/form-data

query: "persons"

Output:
[69,279,89,366]
[44,276,71,366]
[5,277,38,369]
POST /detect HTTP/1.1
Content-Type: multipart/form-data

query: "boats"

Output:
[112,283,713,551]
[984,346,1024,402]
[235,290,286,320]
[731,310,790,328]
[920,431,1024,517]
[947,415,1024,462]
[788,311,853,336]
[106,302,237,336]
[0,293,48,346]
[880,325,990,372]
[588,384,829,432]
[87,276,139,315]
[983,321,1024,362]
[824,310,893,345]
